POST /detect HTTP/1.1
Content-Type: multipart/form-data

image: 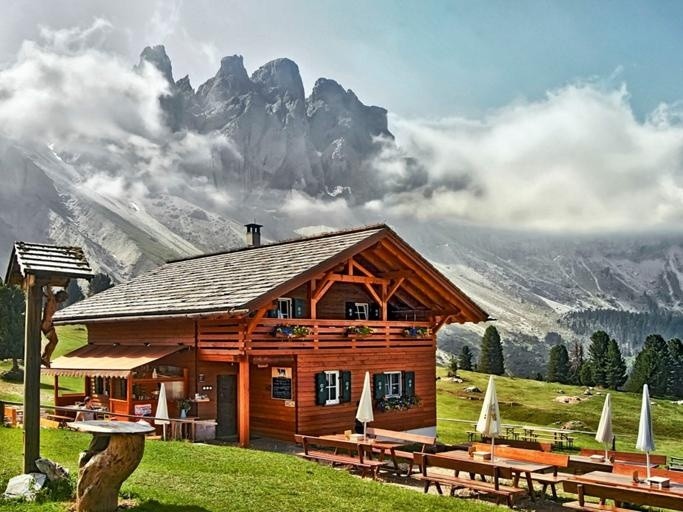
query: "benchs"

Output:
[563,479,683,511]
[581,448,667,469]
[467,424,519,442]
[294,433,388,480]
[472,441,570,499]
[366,427,436,477]
[521,426,575,451]
[611,462,683,485]
[508,440,554,452]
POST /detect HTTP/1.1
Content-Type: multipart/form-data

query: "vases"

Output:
[348,332,373,338]
[275,330,305,339]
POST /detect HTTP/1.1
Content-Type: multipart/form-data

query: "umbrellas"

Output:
[153,383,171,442]
[475,374,501,462]
[594,391,614,460]
[356,369,376,439]
[634,383,657,485]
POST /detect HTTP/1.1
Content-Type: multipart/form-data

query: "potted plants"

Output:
[174,399,192,418]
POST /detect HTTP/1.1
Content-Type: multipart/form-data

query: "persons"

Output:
[40,284,69,368]
[354,400,364,433]
[74,397,96,422]
[137,414,156,435]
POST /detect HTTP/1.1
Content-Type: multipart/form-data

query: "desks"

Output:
[60,419,159,512]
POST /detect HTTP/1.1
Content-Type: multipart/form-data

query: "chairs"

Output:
[413,450,526,510]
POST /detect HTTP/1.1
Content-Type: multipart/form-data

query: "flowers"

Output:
[403,326,428,338]
[345,325,373,337]
[273,322,311,339]
[376,395,423,414]
